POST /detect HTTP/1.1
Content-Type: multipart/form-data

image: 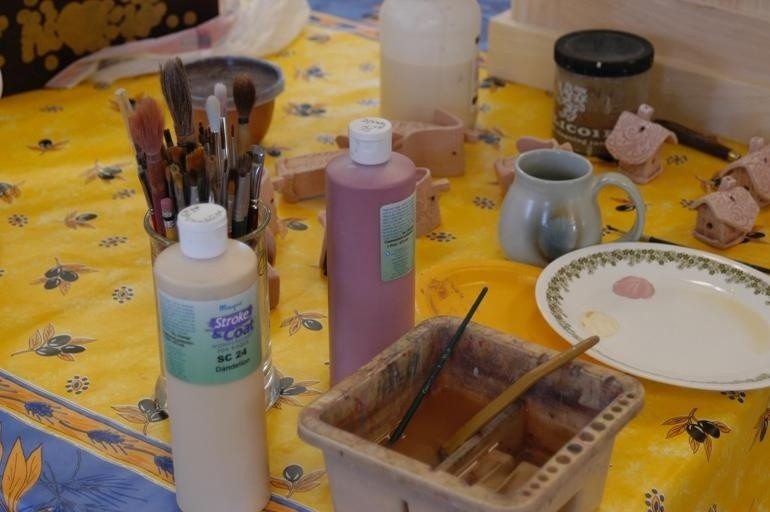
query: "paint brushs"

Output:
[128,58,265,240]
[389,286,489,447]
[439,335,599,464]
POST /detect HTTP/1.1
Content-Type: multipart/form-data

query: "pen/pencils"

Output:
[654,119,741,161]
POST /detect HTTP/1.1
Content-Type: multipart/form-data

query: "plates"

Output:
[535,242,770,391]
[413,256,625,375]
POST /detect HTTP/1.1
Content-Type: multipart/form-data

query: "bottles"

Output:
[380,0,481,138]
[498,147,645,270]
[324,116,417,389]
[551,29,655,158]
[153,202,272,512]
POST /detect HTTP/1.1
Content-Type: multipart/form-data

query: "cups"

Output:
[143,199,282,419]
